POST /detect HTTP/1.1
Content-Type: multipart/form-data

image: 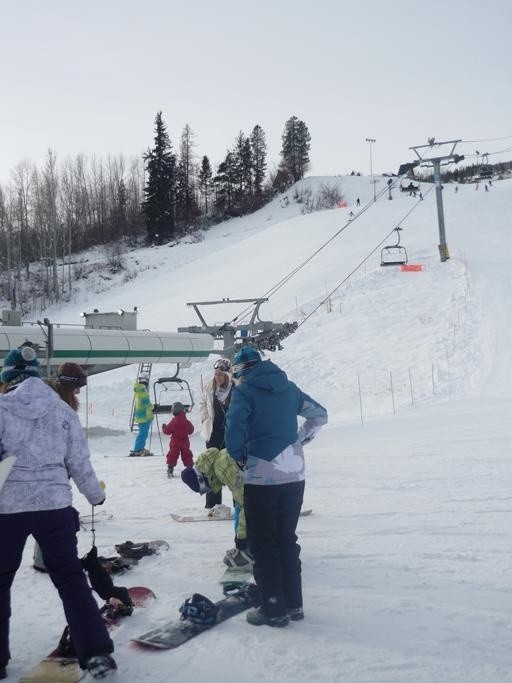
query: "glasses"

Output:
[60,375,88,387]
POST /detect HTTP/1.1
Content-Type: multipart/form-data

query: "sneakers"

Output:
[0,665,7,679]
[267,595,305,621]
[85,653,118,680]
[133,448,150,456]
[246,605,290,628]
[223,550,254,567]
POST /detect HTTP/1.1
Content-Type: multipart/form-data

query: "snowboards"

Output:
[105,540,169,576]
[16,587,156,683]
[131,586,258,650]
[219,548,252,585]
[170,511,313,522]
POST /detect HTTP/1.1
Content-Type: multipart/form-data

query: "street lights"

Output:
[365,138,377,175]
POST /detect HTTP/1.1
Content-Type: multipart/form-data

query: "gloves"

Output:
[235,456,248,471]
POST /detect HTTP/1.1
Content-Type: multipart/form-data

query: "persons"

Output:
[32,362,87,572]
[180,447,254,568]
[348,171,492,217]
[199,358,240,510]
[133,373,155,452]
[225,345,328,628]
[0,339,117,682]
[162,402,193,474]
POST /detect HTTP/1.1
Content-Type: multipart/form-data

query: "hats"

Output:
[57,361,88,378]
[172,402,184,414]
[137,372,150,383]
[0,341,42,383]
[232,347,263,378]
[180,465,210,493]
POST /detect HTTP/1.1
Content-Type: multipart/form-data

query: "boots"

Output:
[167,464,174,473]
